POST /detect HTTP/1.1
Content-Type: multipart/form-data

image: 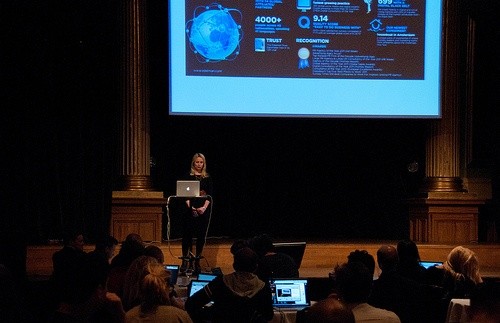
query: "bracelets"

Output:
[203,206,207,210]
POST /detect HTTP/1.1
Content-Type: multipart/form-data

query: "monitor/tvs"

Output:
[165,265,180,272]
[419,261,443,269]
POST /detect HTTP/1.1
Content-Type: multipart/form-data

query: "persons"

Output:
[306,239,500,323]
[186,231,300,323]
[45,229,193,323]
[178,153,215,274]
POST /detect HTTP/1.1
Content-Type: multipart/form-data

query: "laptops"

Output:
[188,273,218,309]
[176,181,200,197]
[267,278,311,311]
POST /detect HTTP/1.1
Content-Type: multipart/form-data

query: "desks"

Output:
[446,298,470,323]
